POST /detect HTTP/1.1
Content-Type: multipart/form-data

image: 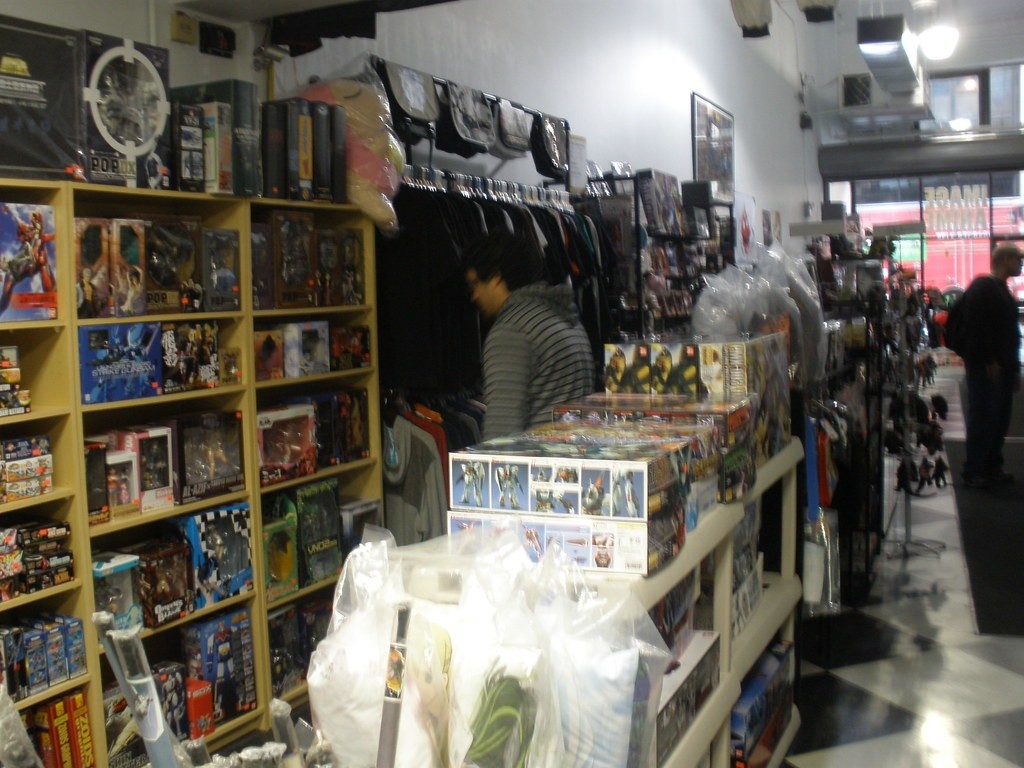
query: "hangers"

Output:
[386,162,585,219]
[376,369,490,438]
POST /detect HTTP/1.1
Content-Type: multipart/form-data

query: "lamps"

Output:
[731,1,773,39]
[798,0,836,24]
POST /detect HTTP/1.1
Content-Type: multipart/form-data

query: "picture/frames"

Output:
[691,91,736,207]
[774,210,782,246]
[762,209,773,246]
[733,191,756,261]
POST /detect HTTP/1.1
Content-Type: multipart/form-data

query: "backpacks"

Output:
[945,274,1006,350]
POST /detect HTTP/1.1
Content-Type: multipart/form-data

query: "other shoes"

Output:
[964,476,992,490]
[986,472,1014,483]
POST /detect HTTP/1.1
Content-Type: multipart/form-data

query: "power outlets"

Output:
[170,11,198,45]
[805,201,814,218]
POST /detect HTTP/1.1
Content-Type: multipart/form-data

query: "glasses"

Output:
[466,276,482,293]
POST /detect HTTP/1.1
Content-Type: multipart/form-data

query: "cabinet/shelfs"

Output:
[693,256,888,621]
[387,438,802,767]
[582,175,738,341]
[0,177,386,768]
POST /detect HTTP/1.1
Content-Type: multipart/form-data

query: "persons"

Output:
[940,243,1024,488]
[464,230,596,441]
